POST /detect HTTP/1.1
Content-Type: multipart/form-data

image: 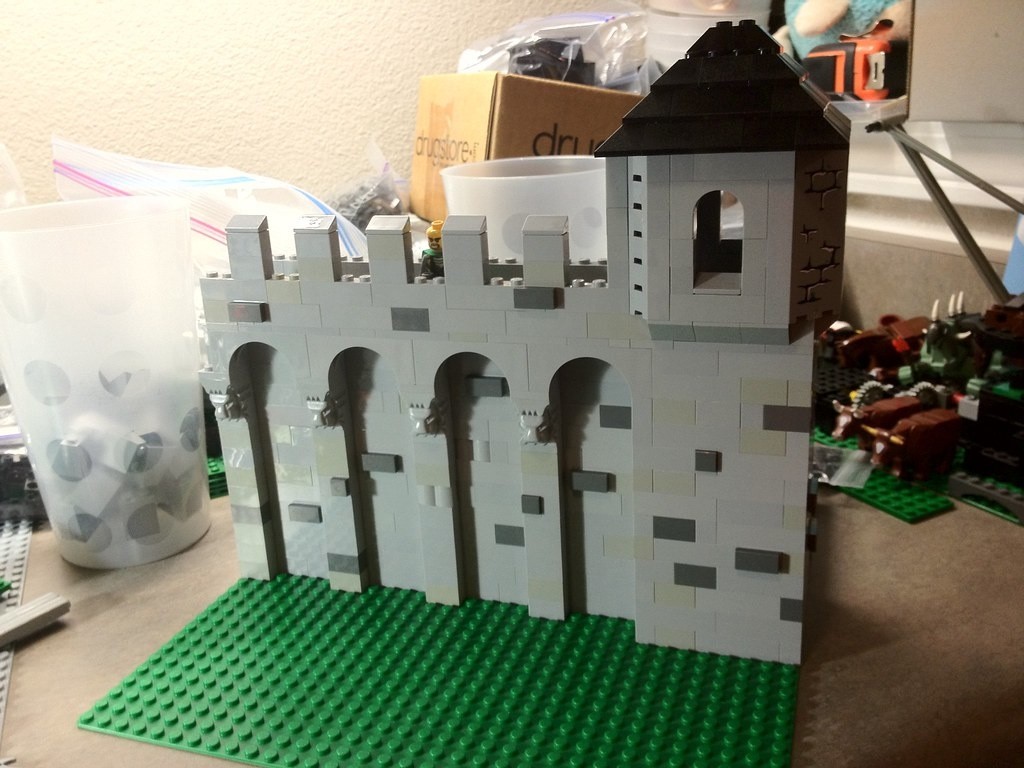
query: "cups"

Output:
[0,195,213,570]
[438,155,606,263]
[646,0,771,74]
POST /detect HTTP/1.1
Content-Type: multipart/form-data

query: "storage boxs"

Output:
[411,70,647,225]
